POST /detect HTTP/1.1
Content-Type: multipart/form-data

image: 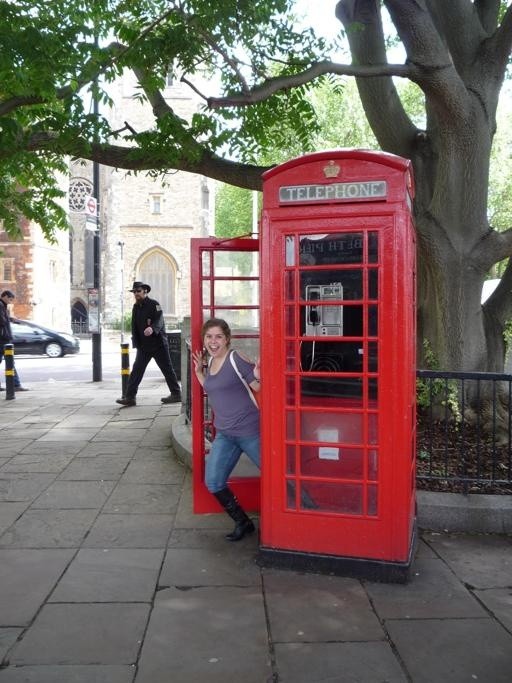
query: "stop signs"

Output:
[86,196,97,216]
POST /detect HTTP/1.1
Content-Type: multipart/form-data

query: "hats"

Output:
[130,282,150,293]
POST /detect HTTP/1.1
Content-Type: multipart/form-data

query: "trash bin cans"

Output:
[167,330,181,383]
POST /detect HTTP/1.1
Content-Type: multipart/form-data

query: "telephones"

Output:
[305,284,343,336]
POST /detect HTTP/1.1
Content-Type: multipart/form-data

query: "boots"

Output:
[212,487,254,541]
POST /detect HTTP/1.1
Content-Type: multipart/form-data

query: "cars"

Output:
[480,277,501,305]
[5,316,80,357]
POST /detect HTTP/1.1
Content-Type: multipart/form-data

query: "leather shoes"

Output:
[161,395,181,403]
[117,400,135,405]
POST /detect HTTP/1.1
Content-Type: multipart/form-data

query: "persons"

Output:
[116,282,181,407]
[191,319,319,542]
[0,291,29,392]
